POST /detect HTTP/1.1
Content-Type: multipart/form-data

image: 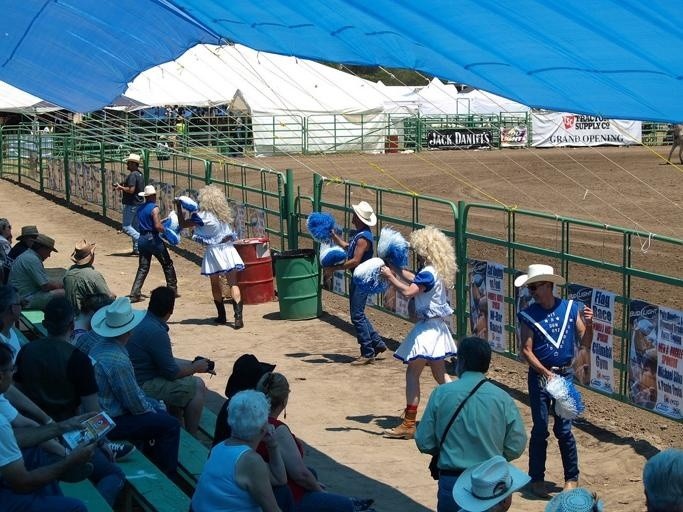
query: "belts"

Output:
[439,469,462,476]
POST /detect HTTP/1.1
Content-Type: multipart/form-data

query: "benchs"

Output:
[0,310,218,511]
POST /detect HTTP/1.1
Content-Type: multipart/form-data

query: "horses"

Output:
[667,122,683,165]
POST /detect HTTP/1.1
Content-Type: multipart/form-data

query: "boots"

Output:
[232,298,243,328]
[214,297,226,323]
[383,409,416,440]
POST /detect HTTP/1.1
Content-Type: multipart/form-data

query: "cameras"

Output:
[191,356,215,371]
[112,183,118,191]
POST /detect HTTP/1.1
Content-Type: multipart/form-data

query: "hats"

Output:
[69,238,96,266]
[225,354,276,398]
[138,184,157,197]
[31,233,57,253]
[545,488,603,512]
[352,201,377,227]
[122,153,143,164]
[16,226,38,240]
[89,296,147,337]
[513,263,566,287]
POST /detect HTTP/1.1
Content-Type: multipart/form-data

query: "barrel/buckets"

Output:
[273,249,322,320]
[385,135,398,153]
[232,237,275,304]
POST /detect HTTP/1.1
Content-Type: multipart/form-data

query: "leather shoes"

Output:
[529,481,548,498]
[562,480,578,492]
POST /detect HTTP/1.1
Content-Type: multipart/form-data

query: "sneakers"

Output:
[350,356,376,365]
[101,439,135,461]
[129,250,139,255]
[129,295,145,303]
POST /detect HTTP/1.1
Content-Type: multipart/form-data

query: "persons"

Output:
[326,201,388,365]
[175,184,245,329]
[513,264,594,498]
[0,217,216,510]
[191,355,374,510]
[545,489,608,512]
[130,185,181,303]
[232,117,243,142]
[115,154,144,256]
[414,336,527,512]
[642,448,683,511]
[155,134,171,161]
[381,224,458,440]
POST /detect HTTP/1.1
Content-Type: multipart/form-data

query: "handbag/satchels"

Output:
[428,447,441,480]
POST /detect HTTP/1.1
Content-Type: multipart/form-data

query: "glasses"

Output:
[527,283,545,291]
[1,365,17,375]
